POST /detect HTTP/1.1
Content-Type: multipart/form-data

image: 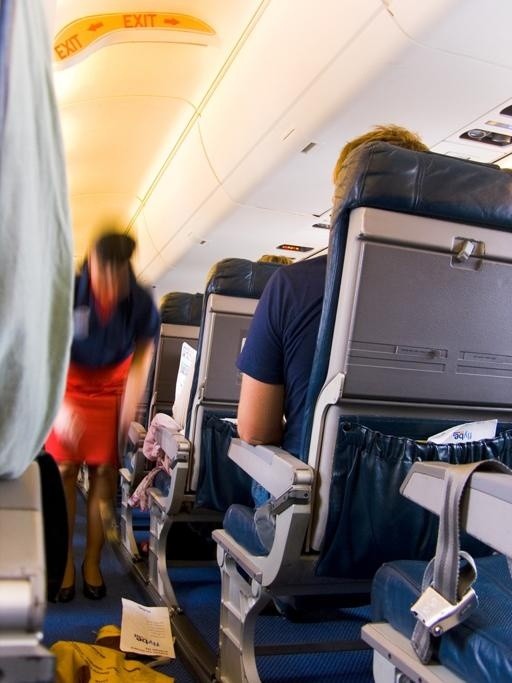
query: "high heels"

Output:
[58,564,76,603]
[81,561,107,600]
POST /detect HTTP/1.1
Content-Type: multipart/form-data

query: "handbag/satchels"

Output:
[95,624,177,664]
[49,640,175,683]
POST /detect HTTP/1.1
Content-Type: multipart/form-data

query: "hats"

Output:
[97,234,135,265]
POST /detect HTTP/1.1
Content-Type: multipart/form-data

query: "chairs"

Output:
[207,139,510,682]
[119,291,203,562]
[143,259,281,612]
[360,458,510,683]
[0,448,69,682]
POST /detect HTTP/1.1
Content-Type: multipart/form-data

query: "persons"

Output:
[238,128,435,507]
[45,232,159,600]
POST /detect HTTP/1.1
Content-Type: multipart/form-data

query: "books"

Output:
[119,597,175,668]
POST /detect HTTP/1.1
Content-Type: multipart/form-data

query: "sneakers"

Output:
[137,540,149,559]
